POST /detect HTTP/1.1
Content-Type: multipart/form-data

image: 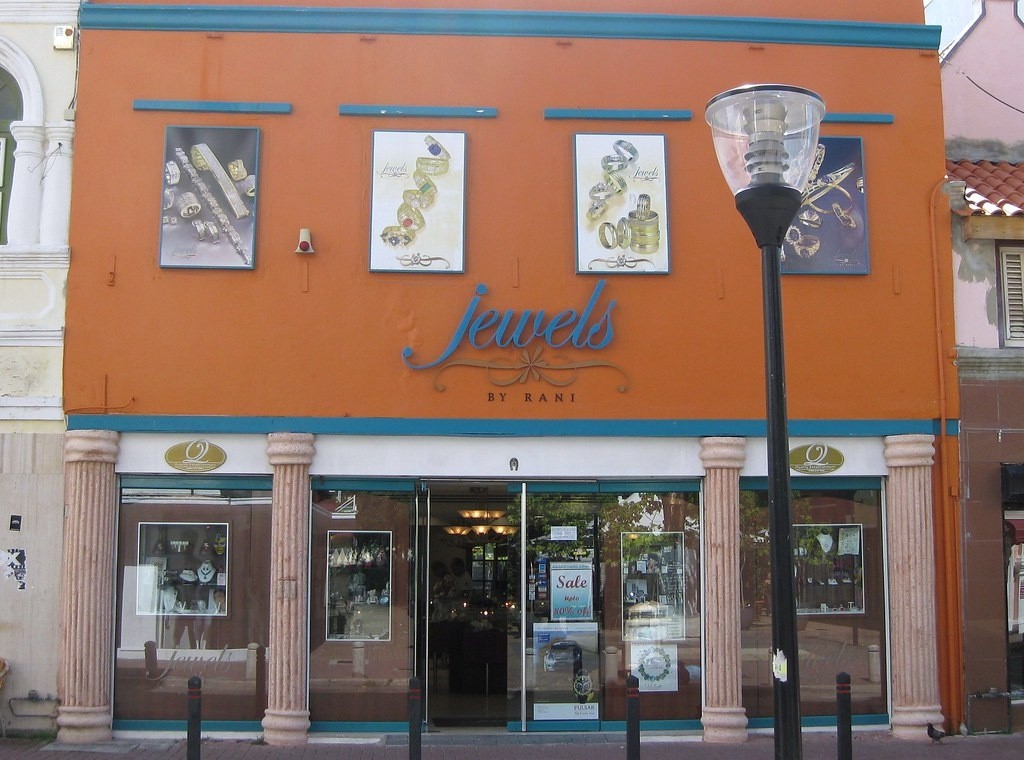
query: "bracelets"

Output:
[638,647,670,682]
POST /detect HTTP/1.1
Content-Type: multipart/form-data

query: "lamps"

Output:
[442,487,520,538]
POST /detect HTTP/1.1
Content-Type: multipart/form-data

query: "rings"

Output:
[785,144,854,259]
[413,171,437,194]
[416,158,449,175]
[599,194,660,253]
[425,136,450,160]
[585,140,640,219]
[397,204,425,230]
[404,190,435,207]
[381,227,415,246]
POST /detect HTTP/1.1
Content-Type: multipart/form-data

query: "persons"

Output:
[164,616,197,650]
[431,559,471,590]
[213,590,226,613]
[632,556,660,594]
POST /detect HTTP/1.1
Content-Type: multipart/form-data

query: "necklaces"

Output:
[182,573,196,579]
[199,567,213,579]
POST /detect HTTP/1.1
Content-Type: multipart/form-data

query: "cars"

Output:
[543,641,582,671]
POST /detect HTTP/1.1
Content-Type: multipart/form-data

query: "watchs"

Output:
[573,647,592,703]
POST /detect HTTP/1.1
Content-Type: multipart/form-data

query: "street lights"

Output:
[702,79,827,760]
